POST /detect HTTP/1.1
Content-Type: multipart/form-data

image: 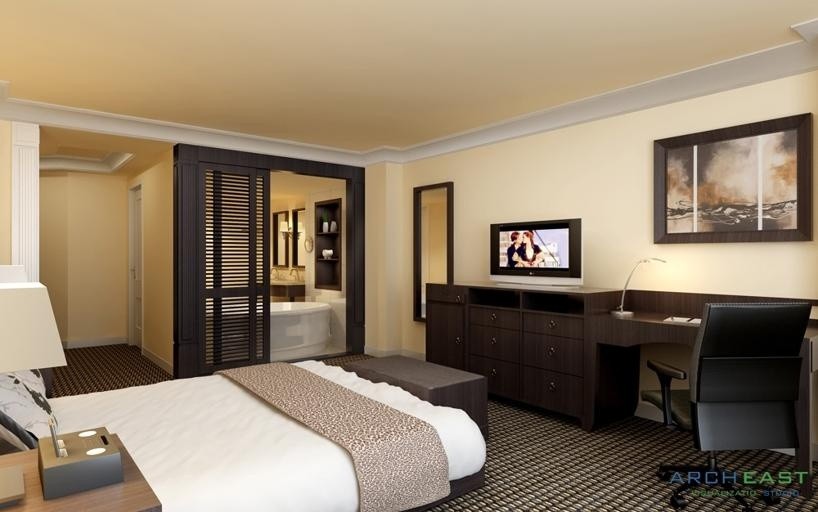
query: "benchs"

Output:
[344,354,489,442]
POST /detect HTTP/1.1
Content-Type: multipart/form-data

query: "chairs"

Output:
[641,302,813,512]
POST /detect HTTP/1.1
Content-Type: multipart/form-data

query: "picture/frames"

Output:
[653,112,813,244]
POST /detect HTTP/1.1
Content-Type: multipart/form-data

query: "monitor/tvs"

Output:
[489,218,584,291]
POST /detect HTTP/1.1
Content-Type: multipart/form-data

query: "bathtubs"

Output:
[205,302,329,362]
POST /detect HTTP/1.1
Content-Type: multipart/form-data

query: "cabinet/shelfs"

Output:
[314,198,342,292]
[423,281,640,431]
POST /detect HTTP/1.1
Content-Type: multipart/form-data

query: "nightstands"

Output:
[0,433,161,512]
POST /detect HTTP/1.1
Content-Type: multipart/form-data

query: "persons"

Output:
[510,231,545,268]
[504,232,523,267]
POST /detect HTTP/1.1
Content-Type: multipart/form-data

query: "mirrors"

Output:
[413,181,454,322]
[272,207,305,268]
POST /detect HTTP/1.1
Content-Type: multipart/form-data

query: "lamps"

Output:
[611,256,667,317]
[0,281,68,373]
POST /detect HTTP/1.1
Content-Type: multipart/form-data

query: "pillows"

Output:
[0,367,58,438]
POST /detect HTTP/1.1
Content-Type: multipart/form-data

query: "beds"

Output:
[47,358,486,512]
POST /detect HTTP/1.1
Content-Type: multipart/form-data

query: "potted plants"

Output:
[320,209,329,233]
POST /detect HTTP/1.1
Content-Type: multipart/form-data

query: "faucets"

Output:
[289,268,300,280]
[269,266,279,278]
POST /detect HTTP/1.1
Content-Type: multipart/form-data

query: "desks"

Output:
[597,288,818,498]
